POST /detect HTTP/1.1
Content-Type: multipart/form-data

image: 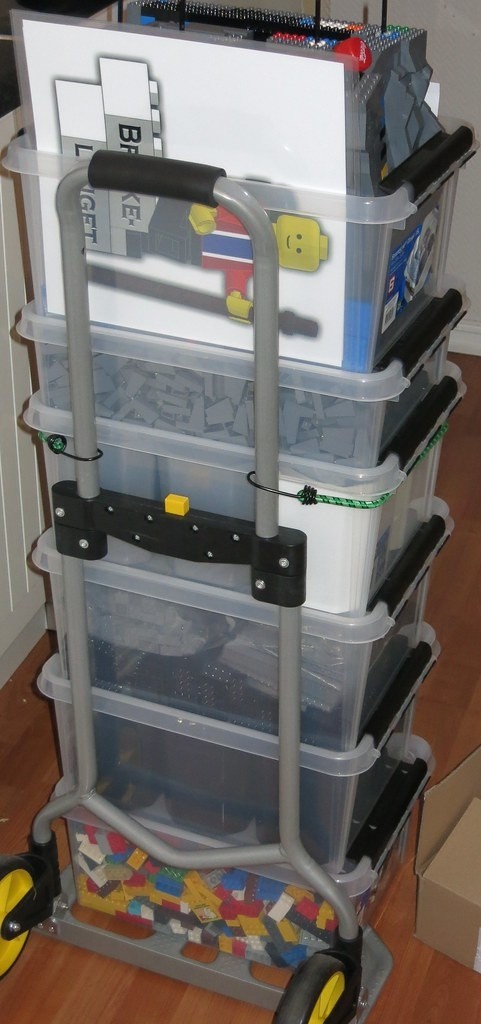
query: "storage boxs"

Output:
[1,118,480,971]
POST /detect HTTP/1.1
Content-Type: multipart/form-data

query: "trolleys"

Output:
[0,152,364,1024]
